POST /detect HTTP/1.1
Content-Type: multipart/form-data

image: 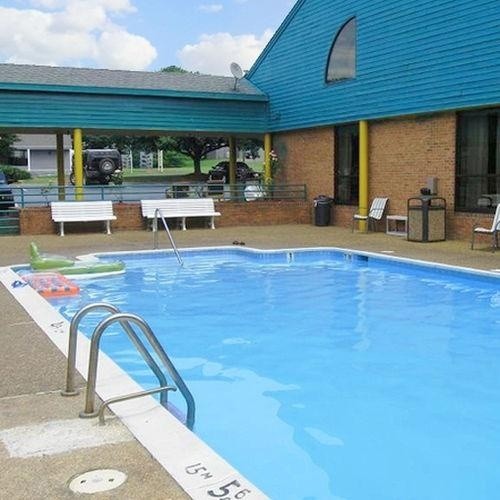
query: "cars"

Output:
[0,172,14,209]
[209,162,252,183]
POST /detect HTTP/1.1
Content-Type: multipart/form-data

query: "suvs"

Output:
[70,148,124,185]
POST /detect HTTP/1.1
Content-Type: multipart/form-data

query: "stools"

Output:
[384,214,408,236]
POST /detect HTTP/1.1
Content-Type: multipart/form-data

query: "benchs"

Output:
[50,200,118,237]
[139,198,222,233]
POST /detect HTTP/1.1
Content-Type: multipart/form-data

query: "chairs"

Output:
[168,179,224,202]
[352,197,388,234]
[471,204,500,251]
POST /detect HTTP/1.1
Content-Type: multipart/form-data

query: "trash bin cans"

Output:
[407,195,446,242]
[314,195,330,226]
